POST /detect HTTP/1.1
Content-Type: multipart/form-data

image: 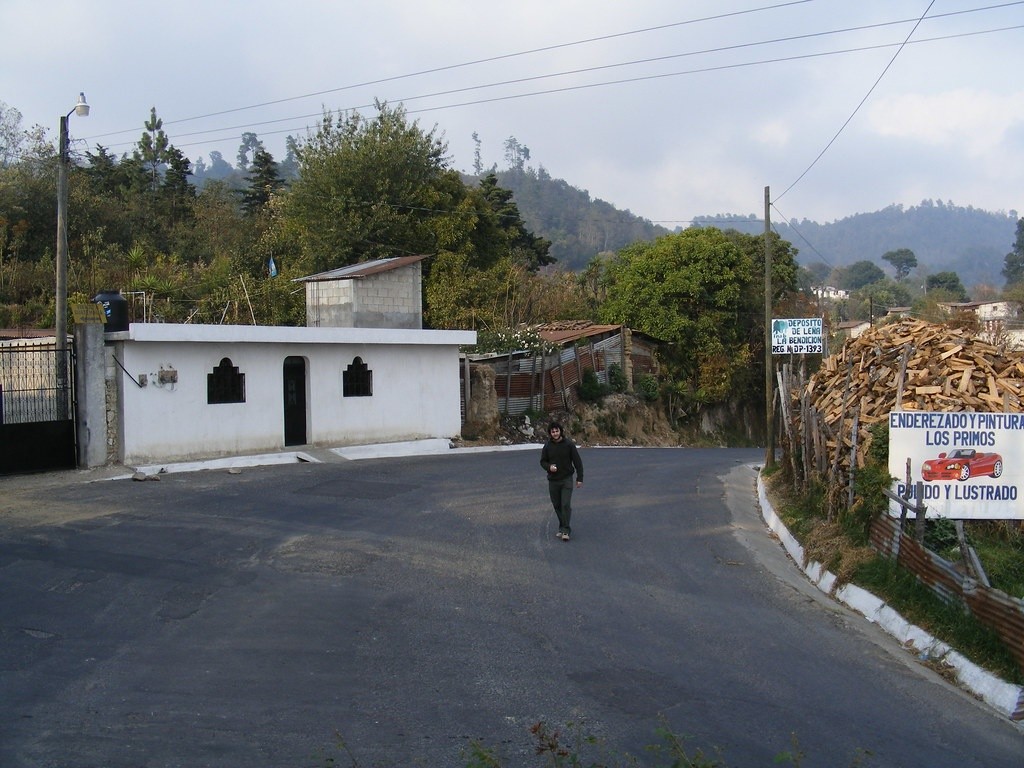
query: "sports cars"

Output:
[920,447,1004,481]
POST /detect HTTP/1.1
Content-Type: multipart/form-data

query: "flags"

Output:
[269,260,277,277]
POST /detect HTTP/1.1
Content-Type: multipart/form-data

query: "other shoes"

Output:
[556,532,562,537]
[562,533,569,539]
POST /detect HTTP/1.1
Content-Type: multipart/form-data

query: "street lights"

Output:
[54,90,92,423]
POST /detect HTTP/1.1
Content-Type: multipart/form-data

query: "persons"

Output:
[540,421,584,541]
